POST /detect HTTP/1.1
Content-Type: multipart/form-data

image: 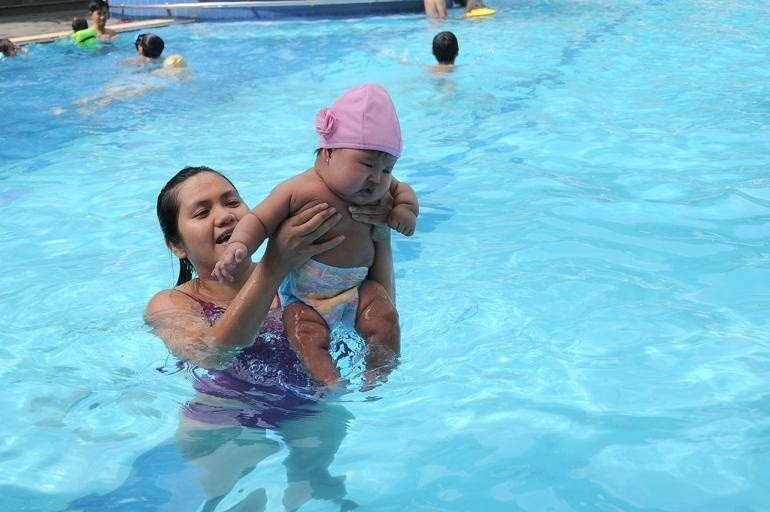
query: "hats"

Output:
[316,84,402,158]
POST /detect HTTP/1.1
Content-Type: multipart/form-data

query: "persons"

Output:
[50,52,196,121]
[139,163,350,511]
[423,0,454,24]
[389,28,476,94]
[120,32,164,75]
[210,80,421,394]
[65,16,100,52]
[0,37,29,61]
[464,1,489,17]
[88,0,122,44]
